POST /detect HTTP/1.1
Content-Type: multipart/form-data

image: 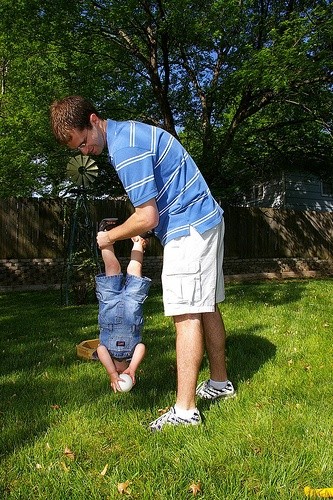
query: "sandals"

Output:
[99,217,155,240]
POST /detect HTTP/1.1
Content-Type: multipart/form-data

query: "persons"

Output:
[51,96,238,430]
[97,218,151,392]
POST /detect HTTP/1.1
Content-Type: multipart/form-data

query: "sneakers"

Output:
[149,380,236,431]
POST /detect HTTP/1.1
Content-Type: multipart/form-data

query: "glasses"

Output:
[72,128,88,152]
